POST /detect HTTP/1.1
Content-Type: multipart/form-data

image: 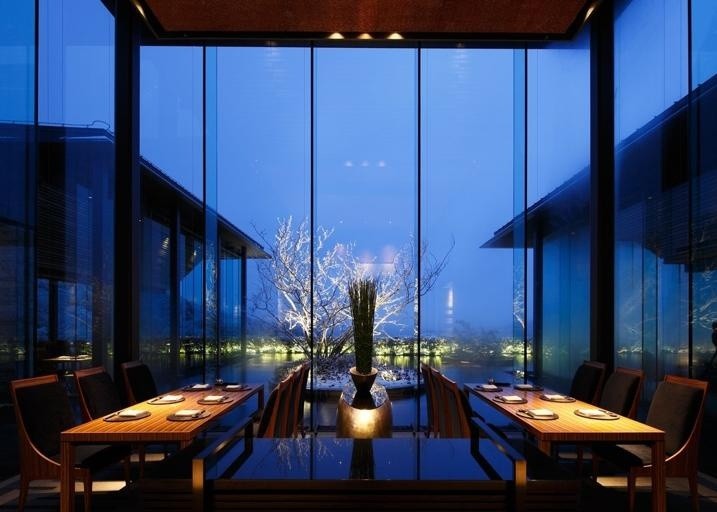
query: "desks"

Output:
[140,438,577,512]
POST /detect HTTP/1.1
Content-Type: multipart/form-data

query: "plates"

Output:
[181,385,212,391]
[196,396,234,405]
[539,394,575,403]
[103,410,150,422]
[474,386,502,393]
[515,408,559,421]
[165,409,211,421]
[575,408,619,421]
[146,395,185,405]
[223,386,252,391]
[492,395,528,405]
[512,384,544,391]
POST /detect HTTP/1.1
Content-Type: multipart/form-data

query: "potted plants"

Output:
[348,281,377,391]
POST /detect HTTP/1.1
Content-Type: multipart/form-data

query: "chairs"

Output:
[10,360,157,512]
[420,362,470,438]
[257,361,310,438]
[551,360,709,512]
